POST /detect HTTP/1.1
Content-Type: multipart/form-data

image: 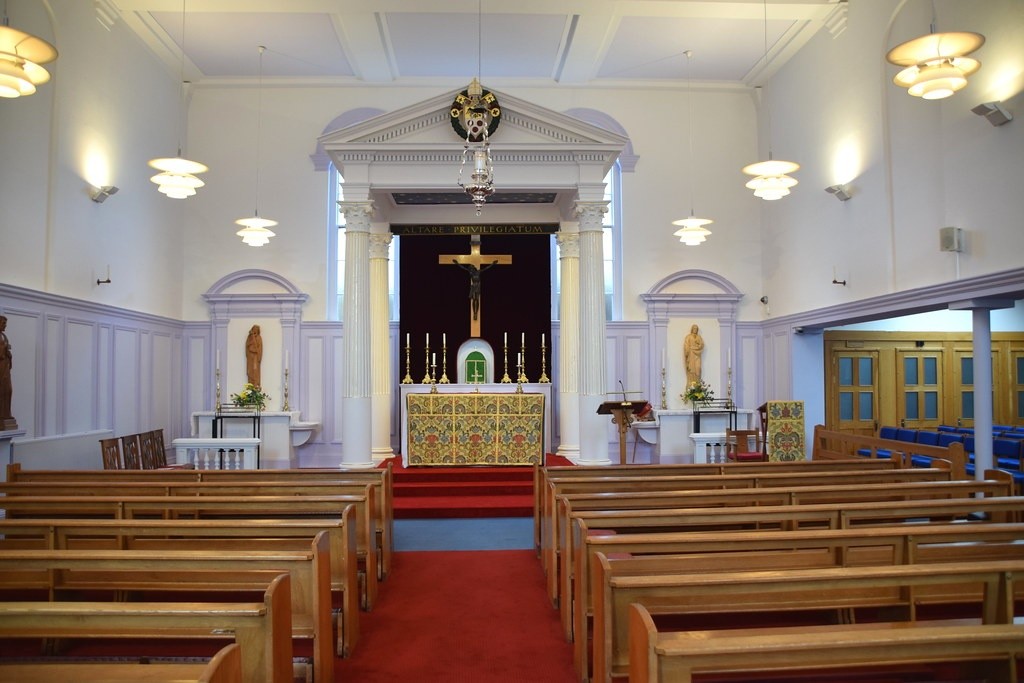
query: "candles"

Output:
[662,349,665,368]
[426,333,429,347]
[216,349,220,370]
[504,332,507,347]
[443,333,446,348]
[517,353,521,365]
[407,333,410,347]
[542,333,545,347]
[432,352,436,365]
[728,348,731,368]
[521,332,524,347]
[285,349,288,369]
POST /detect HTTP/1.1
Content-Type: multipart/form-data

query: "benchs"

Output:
[0,463,394,683]
[533,440,1024,683]
[689,431,769,464]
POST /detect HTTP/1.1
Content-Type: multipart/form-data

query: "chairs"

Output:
[726,426,769,462]
[857,424,1024,486]
[99,429,196,471]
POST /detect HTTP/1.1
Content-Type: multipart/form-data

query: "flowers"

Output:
[681,380,713,404]
[230,383,271,411]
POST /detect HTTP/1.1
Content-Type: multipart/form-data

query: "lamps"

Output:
[886,0,986,100]
[457,0,496,217]
[236,46,278,247]
[672,50,713,247]
[971,99,1013,125]
[93,185,119,203]
[741,0,800,200]
[148,0,208,198]
[0,0,57,98]
[826,184,849,200]
